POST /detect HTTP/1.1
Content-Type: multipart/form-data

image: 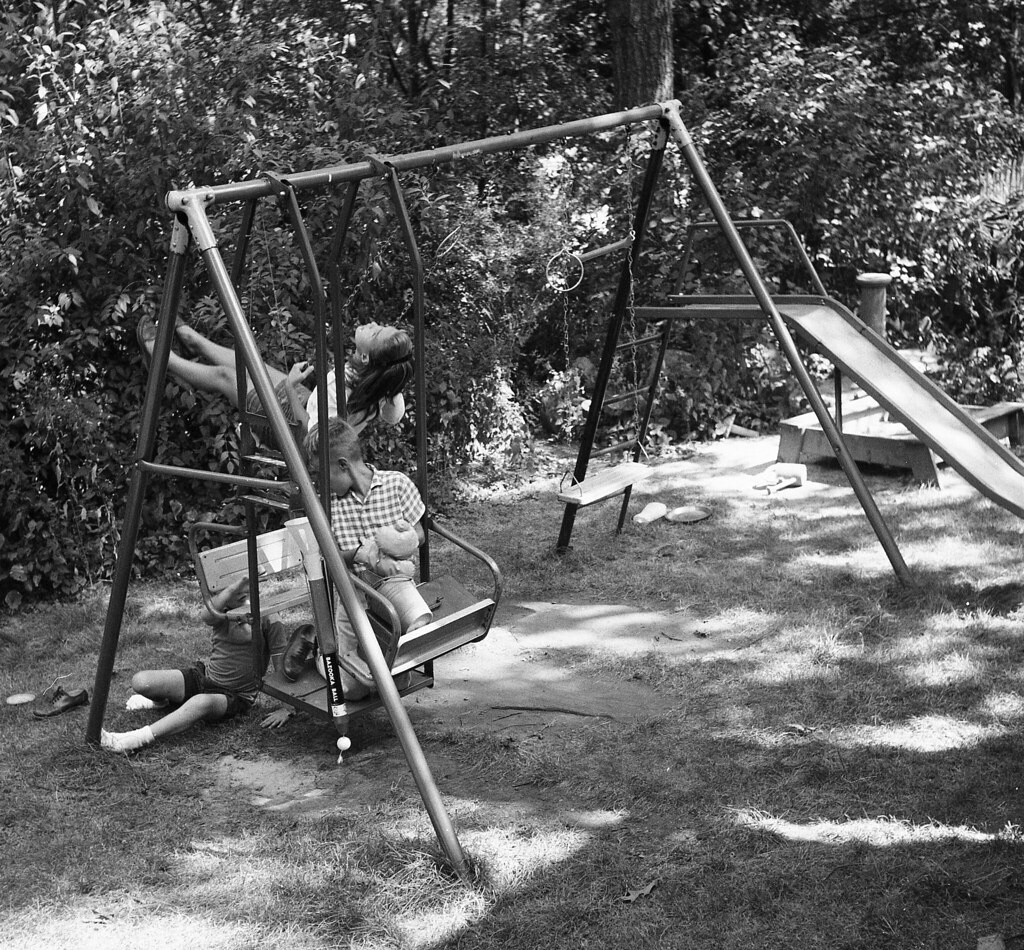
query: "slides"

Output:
[780,296,1024,517]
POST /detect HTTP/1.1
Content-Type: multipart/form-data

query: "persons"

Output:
[100,570,296,753]
[137,315,416,452]
[300,417,426,701]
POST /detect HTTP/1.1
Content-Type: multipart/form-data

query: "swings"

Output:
[552,123,646,506]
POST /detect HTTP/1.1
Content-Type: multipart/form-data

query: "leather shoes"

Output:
[281,624,315,682]
[32,684,88,719]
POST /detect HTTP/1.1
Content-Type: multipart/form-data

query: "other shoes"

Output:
[137,315,156,372]
[172,315,200,359]
